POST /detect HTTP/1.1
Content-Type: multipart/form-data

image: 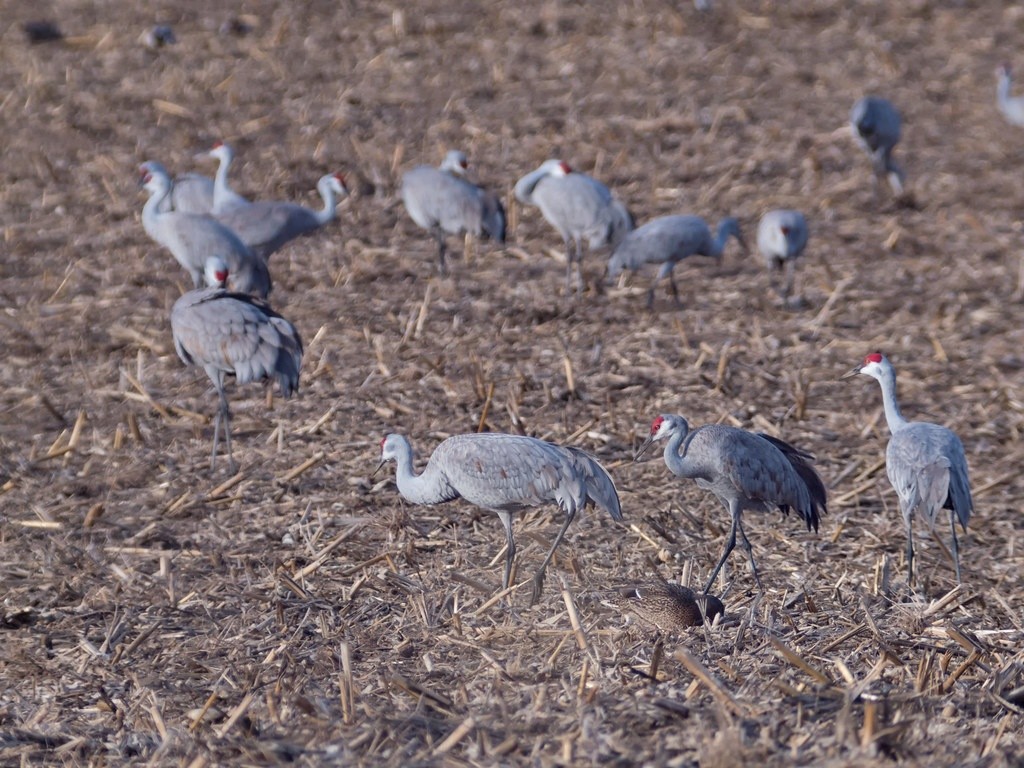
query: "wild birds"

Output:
[619,582,725,648]
[632,414,828,597]
[602,213,751,310]
[990,61,1024,130]
[756,211,808,307]
[397,149,506,272]
[513,159,632,296]
[372,432,623,615]
[172,256,305,473]
[133,141,360,299]
[852,97,907,196]
[837,353,974,589]
